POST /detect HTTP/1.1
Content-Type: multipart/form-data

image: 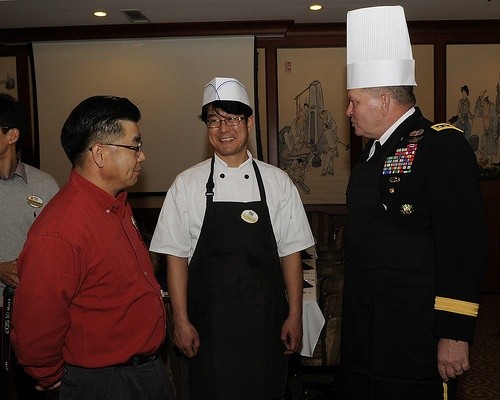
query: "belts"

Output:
[130,350,161,365]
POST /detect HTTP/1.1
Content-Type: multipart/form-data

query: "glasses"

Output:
[203,116,245,128]
[88,141,143,154]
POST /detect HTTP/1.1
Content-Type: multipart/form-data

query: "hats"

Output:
[346,4,417,91]
[200,77,254,119]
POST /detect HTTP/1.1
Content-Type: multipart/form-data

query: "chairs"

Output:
[306,211,344,365]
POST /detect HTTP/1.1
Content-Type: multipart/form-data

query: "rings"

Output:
[183,351,186,354]
[455,368,463,372]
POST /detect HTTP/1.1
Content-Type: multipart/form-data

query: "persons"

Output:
[9,95,182,400]
[338,5,487,400]
[149,77,317,400]
[0,93,62,400]
